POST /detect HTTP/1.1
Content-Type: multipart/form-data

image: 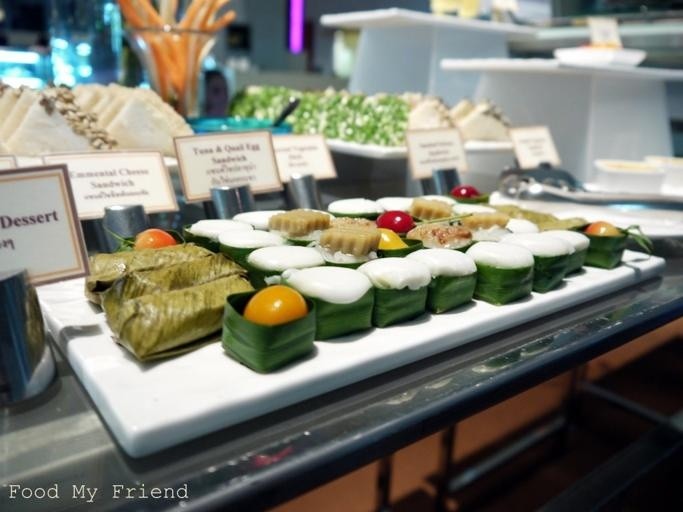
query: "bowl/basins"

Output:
[595,159,663,190]
[643,154,681,184]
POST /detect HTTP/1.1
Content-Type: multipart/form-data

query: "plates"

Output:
[584,178,681,193]
[552,46,647,66]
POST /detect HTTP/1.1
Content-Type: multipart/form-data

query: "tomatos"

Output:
[132,228,175,250]
[242,285,308,326]
[585,221,618,236]
